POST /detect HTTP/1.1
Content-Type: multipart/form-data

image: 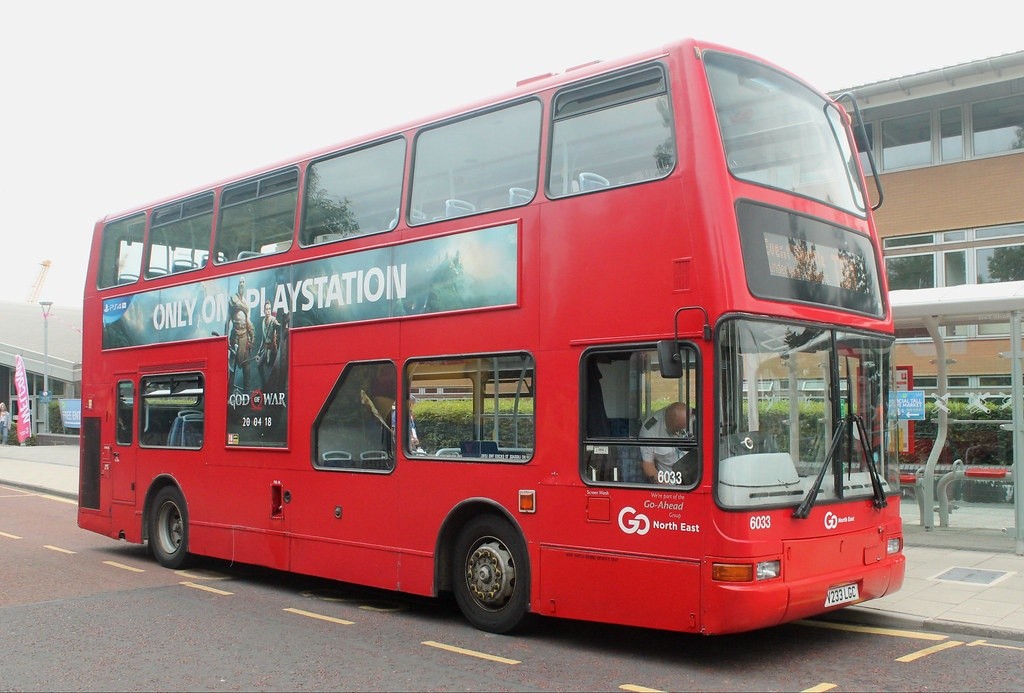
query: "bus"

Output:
[75,37,909,636]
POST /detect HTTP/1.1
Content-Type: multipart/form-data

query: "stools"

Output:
[937,459,1016,527]
[889,467,925,526]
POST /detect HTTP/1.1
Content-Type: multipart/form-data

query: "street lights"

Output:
[38,301,54,435]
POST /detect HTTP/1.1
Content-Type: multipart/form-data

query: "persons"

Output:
[393,396,419,451]
[224,277,289,404]
[0,402,11,446]
[639,402,696,487]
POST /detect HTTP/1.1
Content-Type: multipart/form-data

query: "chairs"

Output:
[322,451,357,468]
[166,410,204,448]
[119,171,610,286]
[360,451,390,469]
